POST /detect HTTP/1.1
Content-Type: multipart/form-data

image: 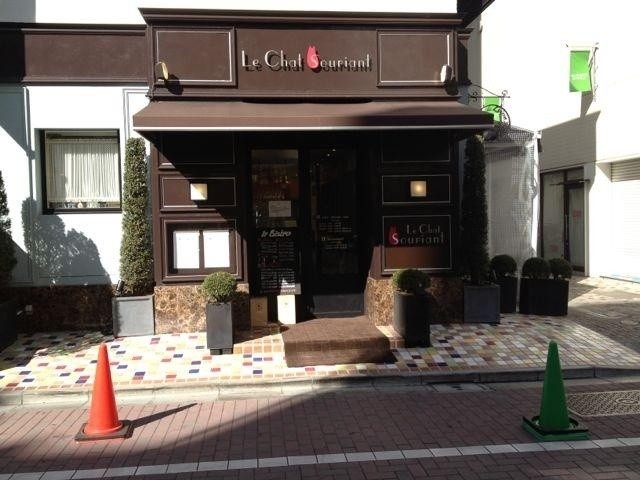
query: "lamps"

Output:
[189,181,210,203]
[408,179,429,199]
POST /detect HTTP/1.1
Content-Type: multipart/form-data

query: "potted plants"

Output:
[111,136,158,338]
[392,267,436,347]
[462,133,573,324]
[199,272,240,355]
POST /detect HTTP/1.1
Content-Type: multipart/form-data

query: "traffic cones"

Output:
[521,340,591,442]
[73,344,134,441]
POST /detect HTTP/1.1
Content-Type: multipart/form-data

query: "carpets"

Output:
[278,315,392,366]
